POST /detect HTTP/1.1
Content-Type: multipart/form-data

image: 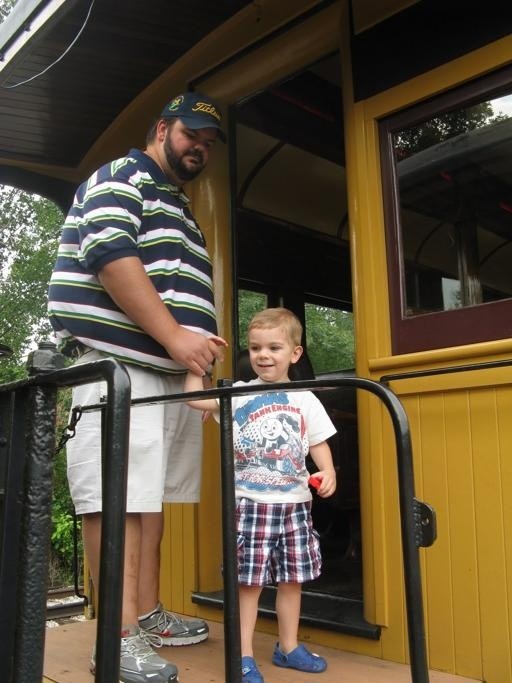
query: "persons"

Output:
[47,91,227,683]
[184,307,337,683]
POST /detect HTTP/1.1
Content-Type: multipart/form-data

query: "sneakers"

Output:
[137,602,209,648]
[89,628,179,683]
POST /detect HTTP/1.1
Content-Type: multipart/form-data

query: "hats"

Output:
[160,91,228,144]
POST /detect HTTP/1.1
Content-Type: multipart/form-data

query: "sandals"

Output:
[272,641,327,673]
[242,655,264,683]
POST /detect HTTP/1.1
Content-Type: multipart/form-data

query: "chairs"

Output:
[234,338,364,585]
